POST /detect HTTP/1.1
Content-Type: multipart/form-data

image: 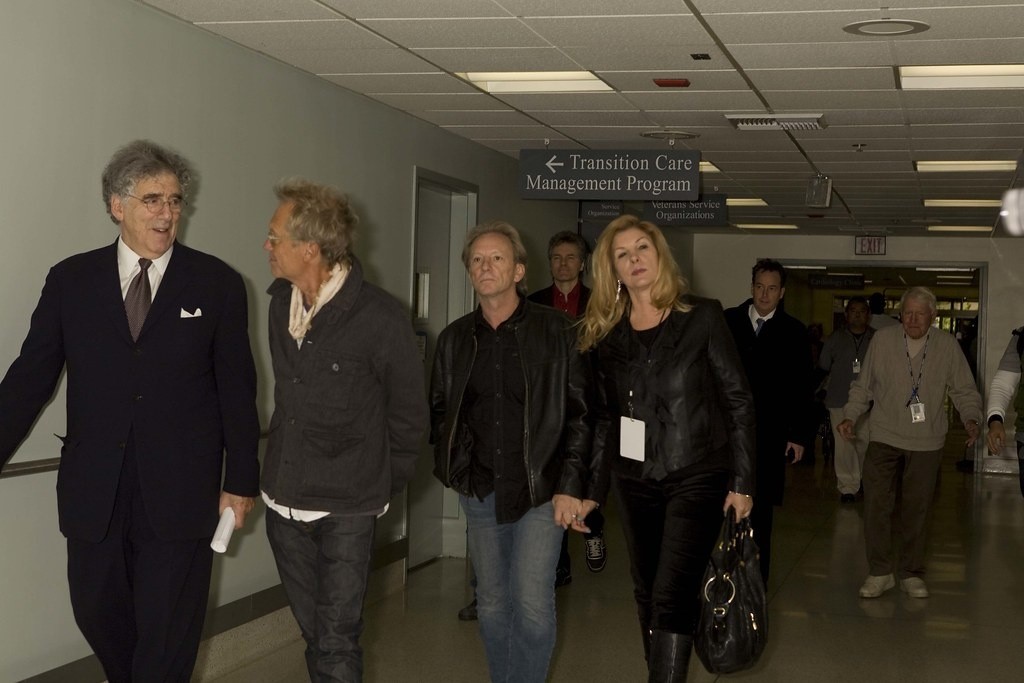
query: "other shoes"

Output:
[855,488,865,502]
[459,600,479,620]
[841,491,854,504]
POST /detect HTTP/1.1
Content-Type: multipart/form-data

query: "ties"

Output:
[124,257,154,343]
[755,318,765,336]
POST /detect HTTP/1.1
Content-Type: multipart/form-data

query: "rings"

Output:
[572,515,577,518]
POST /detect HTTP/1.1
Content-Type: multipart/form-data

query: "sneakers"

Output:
[900,576,929,598]
[584,535,608,572]
[554,569,573,589]
[859,573,896,597]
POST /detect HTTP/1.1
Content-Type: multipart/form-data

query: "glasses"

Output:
[123,192,189,213]
[266,231,302,247]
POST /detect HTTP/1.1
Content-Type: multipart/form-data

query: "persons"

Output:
[808,293,903,506]
[0,139,260,683]
[723,259,814,596]
[525,230,607,588]
[955,330,968,348]
[571,214,754,683]
[428,219,595,683]
[259,178,430,683]
[836,285,984,598]
[458,558,478,621]
[987,324,1024,499]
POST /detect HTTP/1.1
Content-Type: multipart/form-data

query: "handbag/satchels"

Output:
[694,508,769,674]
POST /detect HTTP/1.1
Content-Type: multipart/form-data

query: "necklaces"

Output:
[729,491,752,498]
